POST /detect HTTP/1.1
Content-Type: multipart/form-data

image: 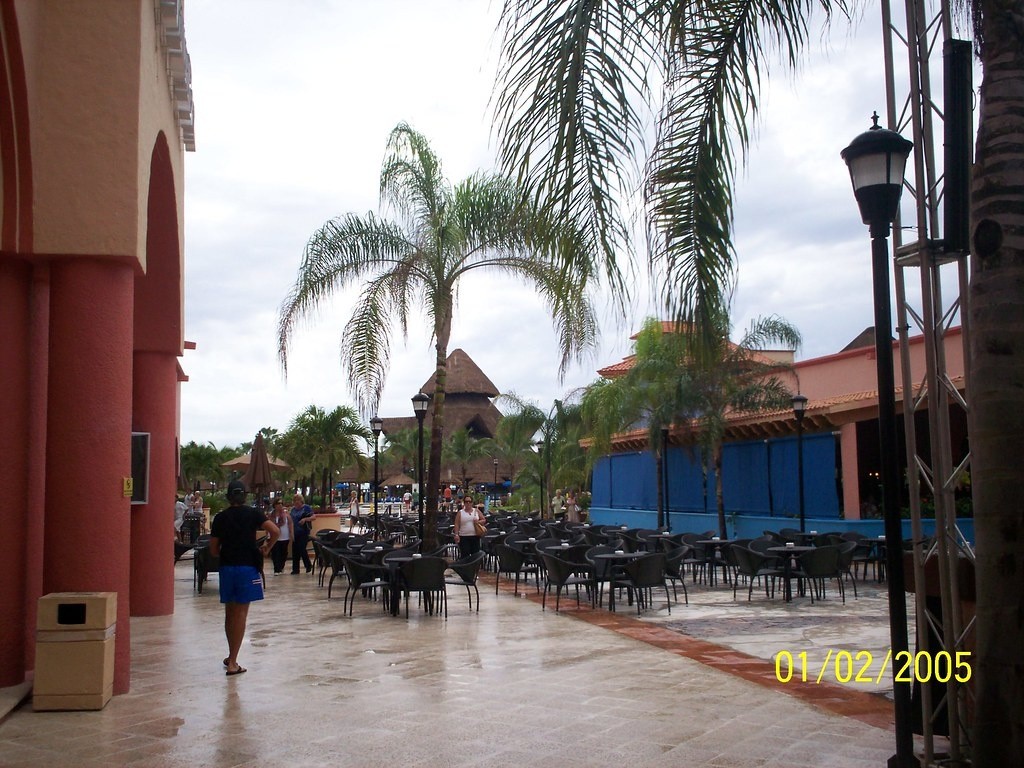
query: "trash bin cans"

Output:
[34,590,115,710]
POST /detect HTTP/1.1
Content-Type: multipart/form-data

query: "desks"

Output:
[696,540,735,587]
[484,534,498,538]
[767,546,816,602]
[386,557,433,617]
[361,550,378,554]
[317,532,327,535]
[573,526,590,529]
[594,552,646,611]
[546,546,570,550]
[648,535,673,539]
[796,533,820,536]
[350,544,363,548]
[861,538,886,583]
[515,540,544,581]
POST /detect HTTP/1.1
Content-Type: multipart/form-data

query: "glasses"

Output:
[276,502,283,505]
[465,501,471,503]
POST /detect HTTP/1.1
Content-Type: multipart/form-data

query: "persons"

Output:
[210,481,281,675]
[348,491,360,534]
[453,496,485,579]
[403,489,418,513]
[263,499,272,512]
[174,488,204,539]
[439,484,464,511]
[291,495,316,574]
[551,488,579,522]
[271,499,292,575]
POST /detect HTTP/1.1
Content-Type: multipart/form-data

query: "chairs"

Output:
[309,512,929,621]
[175,533,269,593]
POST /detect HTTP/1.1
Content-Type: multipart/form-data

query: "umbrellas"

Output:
[333,483,348,488]
[220,434,288,492]
[338,463,507,487]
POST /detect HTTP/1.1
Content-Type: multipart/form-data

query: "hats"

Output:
[225,481,249,495]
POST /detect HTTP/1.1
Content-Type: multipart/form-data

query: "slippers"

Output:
[224,658,238,666]
[226,665,247,675]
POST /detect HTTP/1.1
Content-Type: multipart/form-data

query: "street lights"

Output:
[838,108,924,768]
[791,391,807,542]
[535,438,546,518]
[409,388,432,553]
[493,455,500,508]
[369,413,383,541]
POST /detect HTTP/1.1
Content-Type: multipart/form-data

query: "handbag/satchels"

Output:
[410,496,412,502]
[246,542,264,574]
[474,508,487,536]
[574,497,582,512]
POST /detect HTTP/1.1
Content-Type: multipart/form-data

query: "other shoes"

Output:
[474,574,479,580]
[306,564,312,573]
[274,572,282,576]
[291,570,300,574]
